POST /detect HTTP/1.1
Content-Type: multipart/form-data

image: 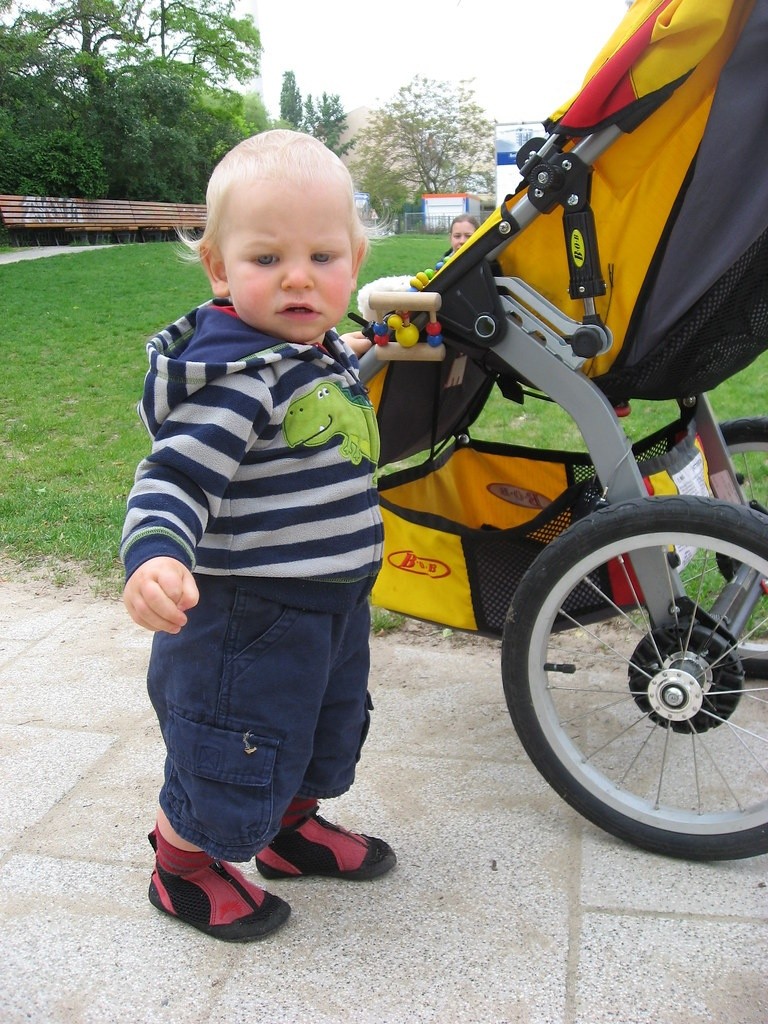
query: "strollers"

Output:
[339,1,767,865]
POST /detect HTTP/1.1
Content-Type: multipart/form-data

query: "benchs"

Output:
[0,194,208,247]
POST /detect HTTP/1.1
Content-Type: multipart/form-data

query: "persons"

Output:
[119,128,398,947]
[434,214,480,272]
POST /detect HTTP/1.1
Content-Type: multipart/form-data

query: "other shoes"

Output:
[256,798,396,881]
[148,821,291,942]
